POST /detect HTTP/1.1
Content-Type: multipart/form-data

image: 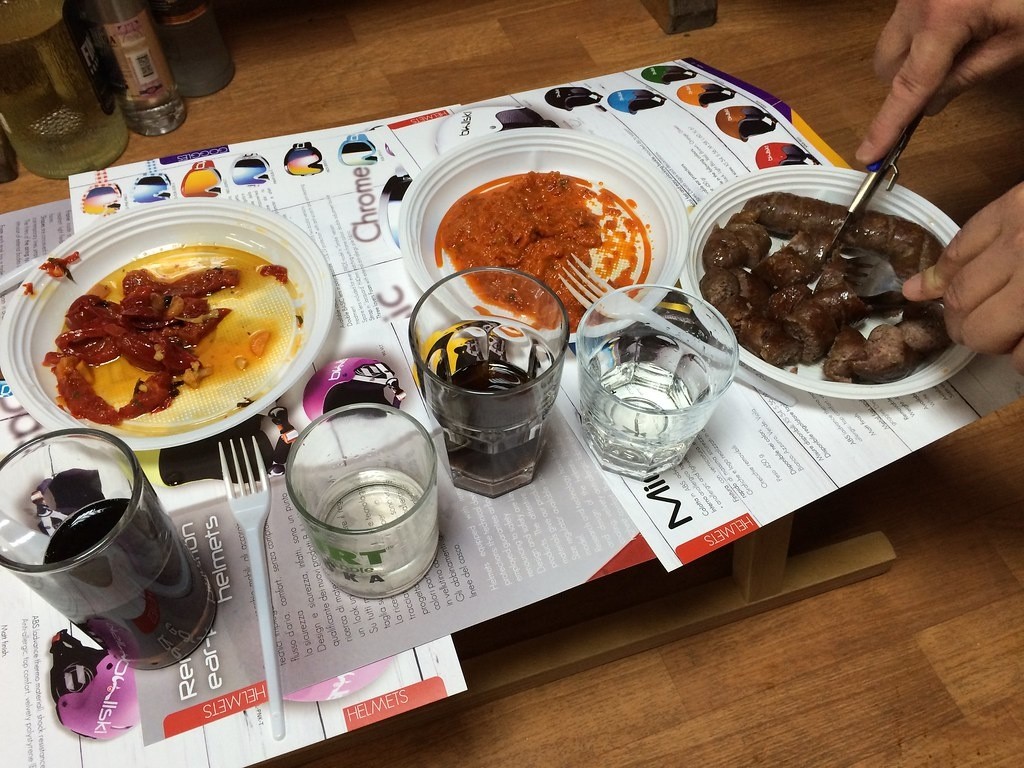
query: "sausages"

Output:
[699,190,953,385]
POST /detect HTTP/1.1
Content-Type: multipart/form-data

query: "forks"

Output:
[559,254,797,407]
[218,435,286,741]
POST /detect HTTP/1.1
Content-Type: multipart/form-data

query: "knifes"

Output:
[825,107,924,261]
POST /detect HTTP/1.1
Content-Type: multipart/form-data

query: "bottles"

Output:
[0,0,234,184]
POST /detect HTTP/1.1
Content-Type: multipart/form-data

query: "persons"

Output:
[852,0,1024,371]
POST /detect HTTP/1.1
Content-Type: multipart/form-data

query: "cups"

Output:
[575,283,740,482]
[0,427,218,672]
[284,402,439,600]
[408,267,570,499]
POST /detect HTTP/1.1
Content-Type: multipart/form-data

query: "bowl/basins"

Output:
[679,163,978,401]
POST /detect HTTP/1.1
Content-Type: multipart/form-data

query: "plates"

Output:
[398,126,691,344]
[0,196,336,451]
